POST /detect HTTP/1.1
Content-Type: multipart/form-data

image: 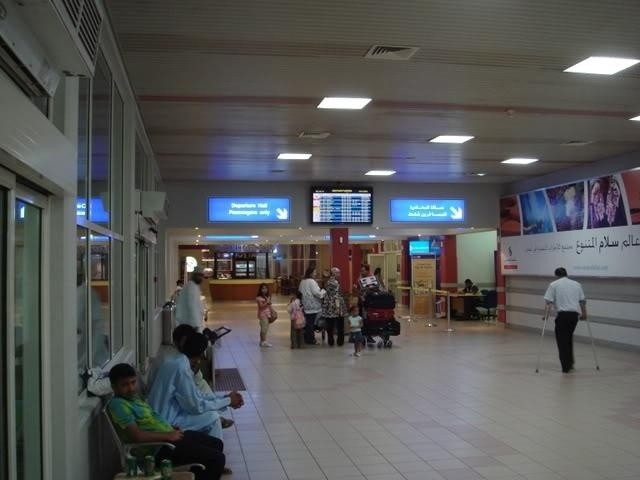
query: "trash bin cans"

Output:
[161,299,176,345]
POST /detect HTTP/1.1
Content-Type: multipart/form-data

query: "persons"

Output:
[257,263,400,355]
[108,267,244,480]
[542,268,587,372]
[459,279,484,317]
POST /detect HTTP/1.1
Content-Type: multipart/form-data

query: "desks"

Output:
[434,291,488,322]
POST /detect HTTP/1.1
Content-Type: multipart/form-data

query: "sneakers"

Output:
[349,351,361,358]
[259,340,272,348]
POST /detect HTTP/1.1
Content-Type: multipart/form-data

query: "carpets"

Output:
[213,366,247,392]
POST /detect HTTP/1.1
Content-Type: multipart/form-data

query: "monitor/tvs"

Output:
[311,185,373,224]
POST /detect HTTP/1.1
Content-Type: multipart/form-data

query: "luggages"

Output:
[358,290,401,337]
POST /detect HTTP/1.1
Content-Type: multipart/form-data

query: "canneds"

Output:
[161,460,172,478]
[125,455,137,478]
[145,455,155,475]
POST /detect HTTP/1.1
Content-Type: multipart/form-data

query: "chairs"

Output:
[480,290,498,321]
[99,392,206,480]
[473,289,489,320]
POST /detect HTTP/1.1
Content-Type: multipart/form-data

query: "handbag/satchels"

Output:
[268,307,280,324]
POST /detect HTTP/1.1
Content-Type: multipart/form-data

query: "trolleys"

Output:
[358,286,401,348]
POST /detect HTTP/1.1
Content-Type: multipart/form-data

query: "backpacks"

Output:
[292,301,306,331]
